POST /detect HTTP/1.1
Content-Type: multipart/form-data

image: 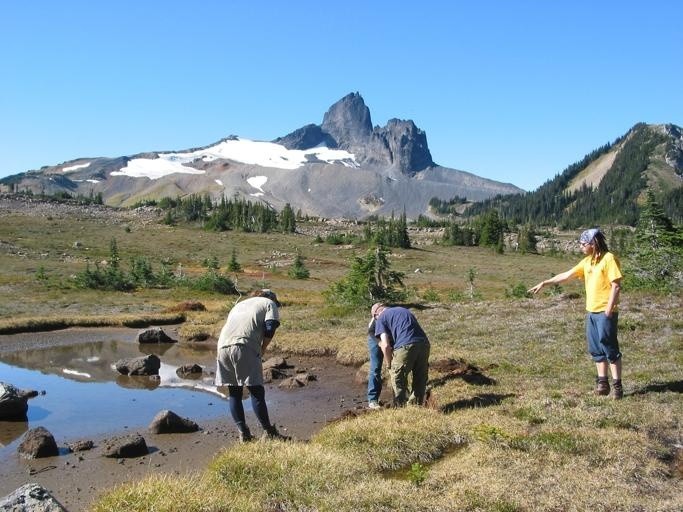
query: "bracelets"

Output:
[542,281,544,288]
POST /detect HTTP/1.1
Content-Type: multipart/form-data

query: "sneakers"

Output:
[239,428,255,444]
[586,383,622,400]
[261,424,291,442]
[368,401,380,409]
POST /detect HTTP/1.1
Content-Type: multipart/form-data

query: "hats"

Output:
[258,288,281,307]
[371,303,383,320]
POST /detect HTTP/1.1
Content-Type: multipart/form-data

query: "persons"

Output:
[525,228,624,401]
[215,288,292,444]
[368,301,430,406]
[367,318,385,408]
